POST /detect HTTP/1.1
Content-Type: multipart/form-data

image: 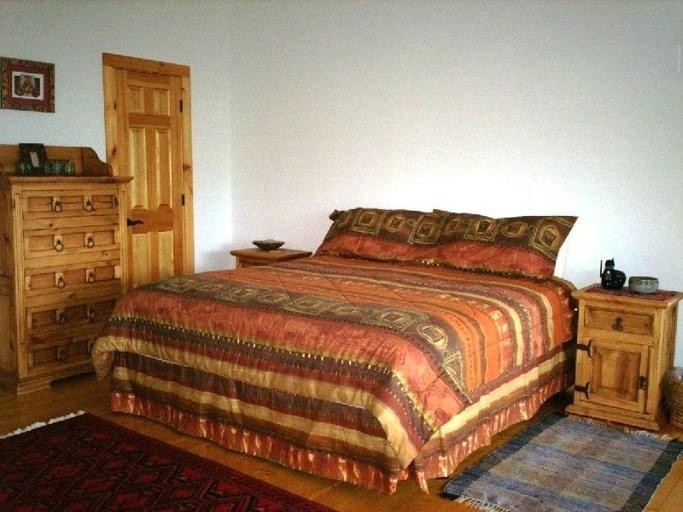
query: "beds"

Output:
[110,256,575,494]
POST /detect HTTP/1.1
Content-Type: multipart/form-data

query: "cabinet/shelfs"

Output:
[1,143,134,398]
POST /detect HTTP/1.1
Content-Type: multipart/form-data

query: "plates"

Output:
[253,240,284,249]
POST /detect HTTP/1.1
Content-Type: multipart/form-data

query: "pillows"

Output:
[311,206,580,284]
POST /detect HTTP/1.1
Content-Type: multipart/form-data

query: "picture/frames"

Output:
[0,56,55,113]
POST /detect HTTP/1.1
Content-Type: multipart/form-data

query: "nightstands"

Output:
[565,281,682,431]
[228,247,317,270]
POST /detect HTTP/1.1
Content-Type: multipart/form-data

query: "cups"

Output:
[45,159,76,175]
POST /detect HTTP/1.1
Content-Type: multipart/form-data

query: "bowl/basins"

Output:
[630,277,658,293]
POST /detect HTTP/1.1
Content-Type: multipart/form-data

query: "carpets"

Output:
[0,406,337,510]
[443,413,683,511]
